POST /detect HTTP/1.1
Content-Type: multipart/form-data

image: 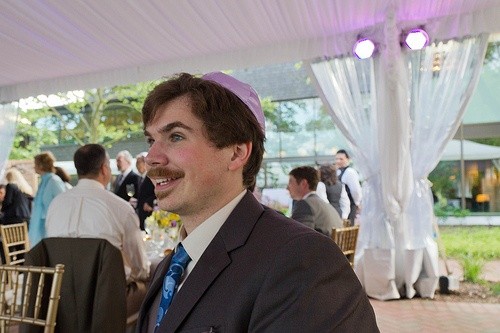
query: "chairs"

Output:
[18,235,148,333]
[0,220,30,290]
[0,259,66,333]
[331,222,361,271]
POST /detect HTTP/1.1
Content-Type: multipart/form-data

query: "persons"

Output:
[136,71,382,333]
[0,167,35,287]
[136,152,157,234]
[315,163,351,227]
[334,149,362,226]
[45,143,151,333]
[114,149,138,202]
[27,152,73,250]
[285,166,343,237]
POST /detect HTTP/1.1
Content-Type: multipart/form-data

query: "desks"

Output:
[141,233,180,277]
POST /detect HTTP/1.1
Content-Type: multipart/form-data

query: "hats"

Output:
[202,72,265,137]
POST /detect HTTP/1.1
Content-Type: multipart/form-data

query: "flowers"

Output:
[144,209,183,241]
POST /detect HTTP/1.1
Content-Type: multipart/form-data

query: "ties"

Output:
[154,242,192,333]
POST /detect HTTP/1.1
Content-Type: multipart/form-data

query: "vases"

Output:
[148,221,180,244]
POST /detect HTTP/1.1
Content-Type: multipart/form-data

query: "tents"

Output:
[254,130,500,219]
[0,0,500,302]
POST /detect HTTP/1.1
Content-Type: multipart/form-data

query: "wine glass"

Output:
[150,229,163,258]
[126,184,135,198]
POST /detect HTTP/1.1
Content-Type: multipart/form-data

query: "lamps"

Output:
[399,25,430,51]
[351,33,380,61]
